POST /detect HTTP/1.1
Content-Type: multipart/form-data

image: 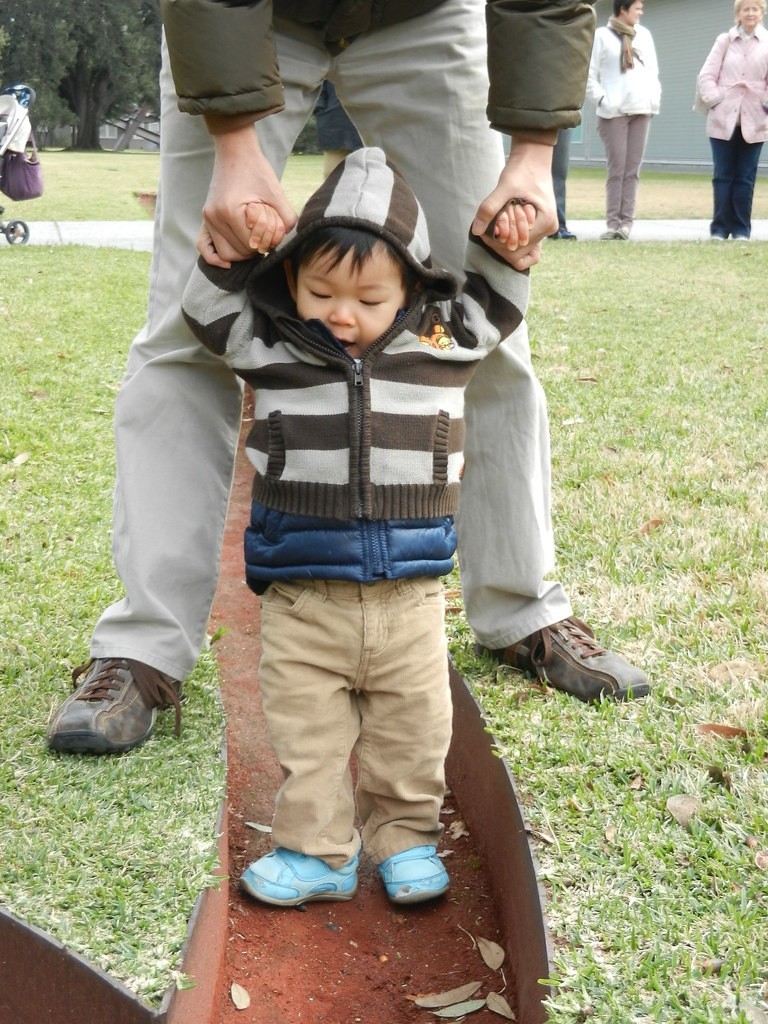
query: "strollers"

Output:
[0,83,38,247]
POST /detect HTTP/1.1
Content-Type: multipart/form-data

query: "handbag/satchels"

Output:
[1,152,43,201]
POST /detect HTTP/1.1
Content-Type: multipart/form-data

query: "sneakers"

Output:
[379,845,450,903]
[238,848,357,905]
[49,659,183,749]
[551,229,575,241]
[478,609,652,703]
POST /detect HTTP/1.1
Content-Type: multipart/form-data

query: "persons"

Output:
[549,126,577,241]
[178,188,553,902]
[52,0,648,764]
[692,1,768,241]
[588,0,662,240]
[314,77,362,179]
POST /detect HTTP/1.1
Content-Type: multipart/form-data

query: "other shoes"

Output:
[712,232,749,241]
[605,228,628,241]
